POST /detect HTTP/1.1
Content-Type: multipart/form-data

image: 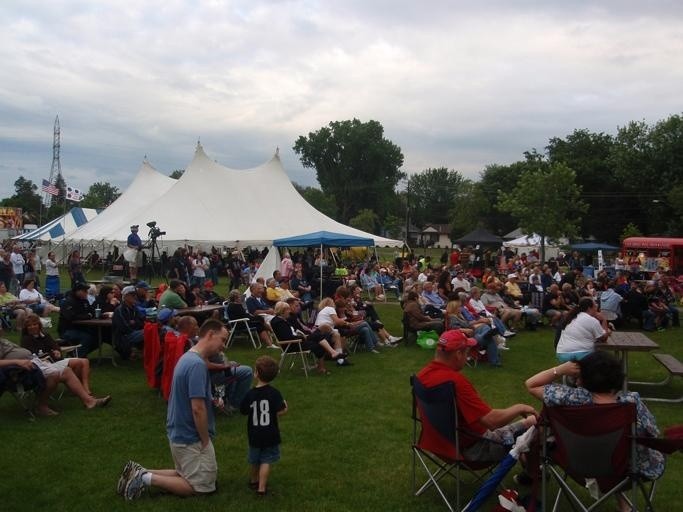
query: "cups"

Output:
[94,307,101,319]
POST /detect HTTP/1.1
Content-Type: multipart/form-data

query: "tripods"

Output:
[144,238,170,285]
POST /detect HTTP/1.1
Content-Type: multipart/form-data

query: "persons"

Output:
[405,242,683,368]
[238,355,288,497]
[524,350,665,512]
[0,226,253,416]
[217,241,403,375]
[414,331,551,486]
[117,319,228,500]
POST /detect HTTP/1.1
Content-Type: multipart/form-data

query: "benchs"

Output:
[629,352,682,402]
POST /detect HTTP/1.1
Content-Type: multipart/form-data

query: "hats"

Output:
[507,274,518,279]
[487,283,497,290]
[72,281,91,292]
[157,308,177,323]
[436,328,478,353]
[136,280,151,289]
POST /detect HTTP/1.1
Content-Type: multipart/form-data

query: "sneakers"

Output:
[116,460,140,494]
[369,349,380,354]
[503,329,516,337]
[124,465,148,503]
[386,340,398,349]
[390,335,404,344]
[513,468,549,486]
[376,341,384,346]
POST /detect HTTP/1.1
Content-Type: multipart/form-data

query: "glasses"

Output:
[108,291,116,294]
[129,293,138,295]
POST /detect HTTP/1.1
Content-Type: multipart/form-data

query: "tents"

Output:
[273,229,386,304]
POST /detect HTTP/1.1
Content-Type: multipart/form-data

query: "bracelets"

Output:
[553,367,559,378]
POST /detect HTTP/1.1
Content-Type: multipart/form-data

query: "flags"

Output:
[42,178,59,196]
[66,186,84,202]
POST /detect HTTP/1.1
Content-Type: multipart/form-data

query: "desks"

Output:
[594,331,660,402]
[73,303,223,365]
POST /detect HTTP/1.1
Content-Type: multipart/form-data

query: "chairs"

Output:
[404,375,683,512]
[0,344,82,423]
[0,295,55,332]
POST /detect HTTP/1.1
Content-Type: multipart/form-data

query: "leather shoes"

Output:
[266,344,280,349]
[336,360,354,367]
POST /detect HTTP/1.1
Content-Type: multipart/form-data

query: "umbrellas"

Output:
[459,424,552,511]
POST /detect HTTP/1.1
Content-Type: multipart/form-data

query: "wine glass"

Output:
[214,383,227,405]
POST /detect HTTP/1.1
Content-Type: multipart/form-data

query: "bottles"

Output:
[33,350,44,359]
[195,294,209,308]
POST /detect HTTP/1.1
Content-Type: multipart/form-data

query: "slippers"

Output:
[331,352,349,360]
[34,407,60,419]
[83,394,112,410]
